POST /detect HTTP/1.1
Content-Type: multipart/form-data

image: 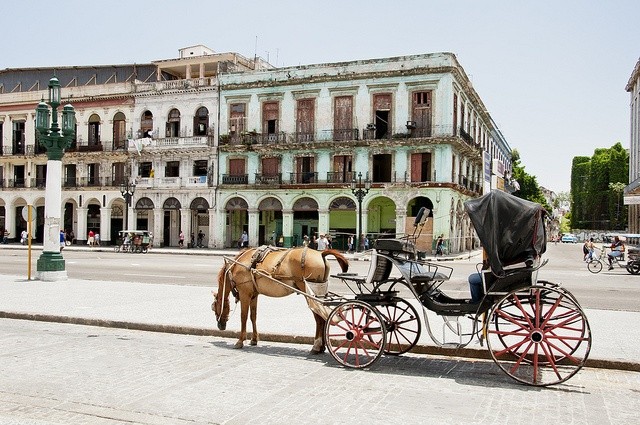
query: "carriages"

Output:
[211,190,592,388]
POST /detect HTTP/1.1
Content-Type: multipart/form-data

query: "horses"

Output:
[211,246,350,355]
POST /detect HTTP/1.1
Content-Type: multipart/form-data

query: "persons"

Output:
[143,128,157,138]
[196,230,205,249]
[21,229,28,245]
[302,235,311,247]
[94,232,101,247]
[586,237,599,263]
[60,229,66,252]
[272,230,277,244]
[240,230,247,248]
[178,230,185,248]
[345,233,355,254]
[465,247,490,304]
[582,241,590,262]
[436,234,445,255]
[3,228,10,244]
[603,236,623,271]
[89,228,94,247]
[361,234,369,251]
[278,234,283,247]
[118,232,154,251]
[313,232,329,252]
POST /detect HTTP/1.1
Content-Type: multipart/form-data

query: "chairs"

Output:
[332,207,453,298]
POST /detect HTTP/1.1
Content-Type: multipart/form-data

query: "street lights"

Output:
[121,176,135,230]
[351,172,372,251]
[34,77,76,281]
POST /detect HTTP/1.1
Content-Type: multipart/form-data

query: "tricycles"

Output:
[590,233,640,275]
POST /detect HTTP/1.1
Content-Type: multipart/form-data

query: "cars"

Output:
[562,233,578,243]
